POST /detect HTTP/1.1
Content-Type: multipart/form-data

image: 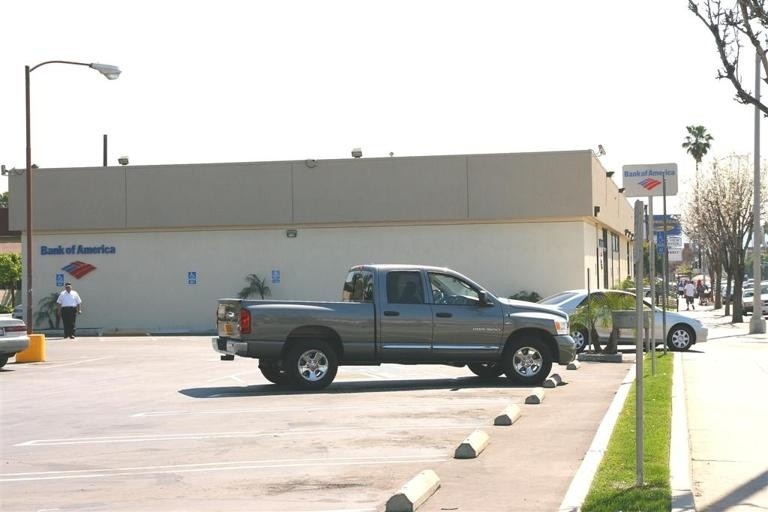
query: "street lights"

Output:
[21,58,131,336]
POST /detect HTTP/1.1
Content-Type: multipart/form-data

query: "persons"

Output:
[55,282,83,339]
[696,281,708,306]
[684,280,697,310]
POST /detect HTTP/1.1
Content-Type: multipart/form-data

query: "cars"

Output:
[0,317,31,373]
[10,304,25,319]
[525,288,710,355]
[675,275,768,319]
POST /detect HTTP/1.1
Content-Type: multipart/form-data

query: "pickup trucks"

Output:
[210,262,576,391]
